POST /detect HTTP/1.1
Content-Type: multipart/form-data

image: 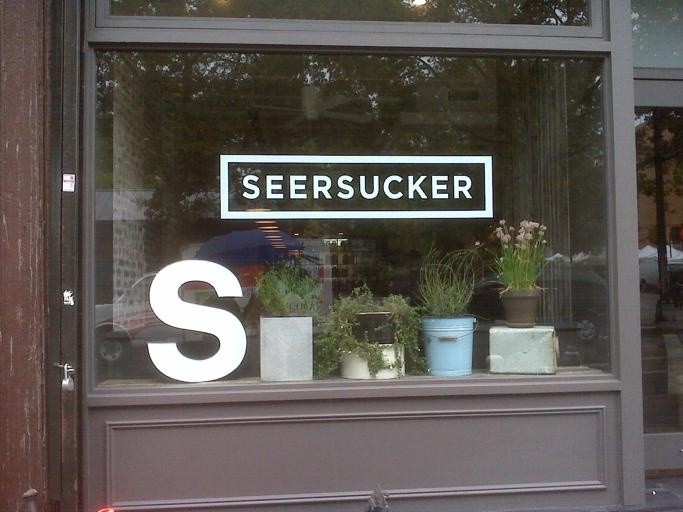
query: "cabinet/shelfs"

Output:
[295,236,381,293]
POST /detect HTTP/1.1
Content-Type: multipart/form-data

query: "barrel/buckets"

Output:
[420,317,478,377]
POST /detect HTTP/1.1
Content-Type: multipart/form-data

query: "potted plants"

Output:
[313,283,428,380]
[501,293,540,328]
[417,248,481,376]
[257,265,321,380]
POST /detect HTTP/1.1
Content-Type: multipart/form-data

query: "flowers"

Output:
[475,219,548,292]
